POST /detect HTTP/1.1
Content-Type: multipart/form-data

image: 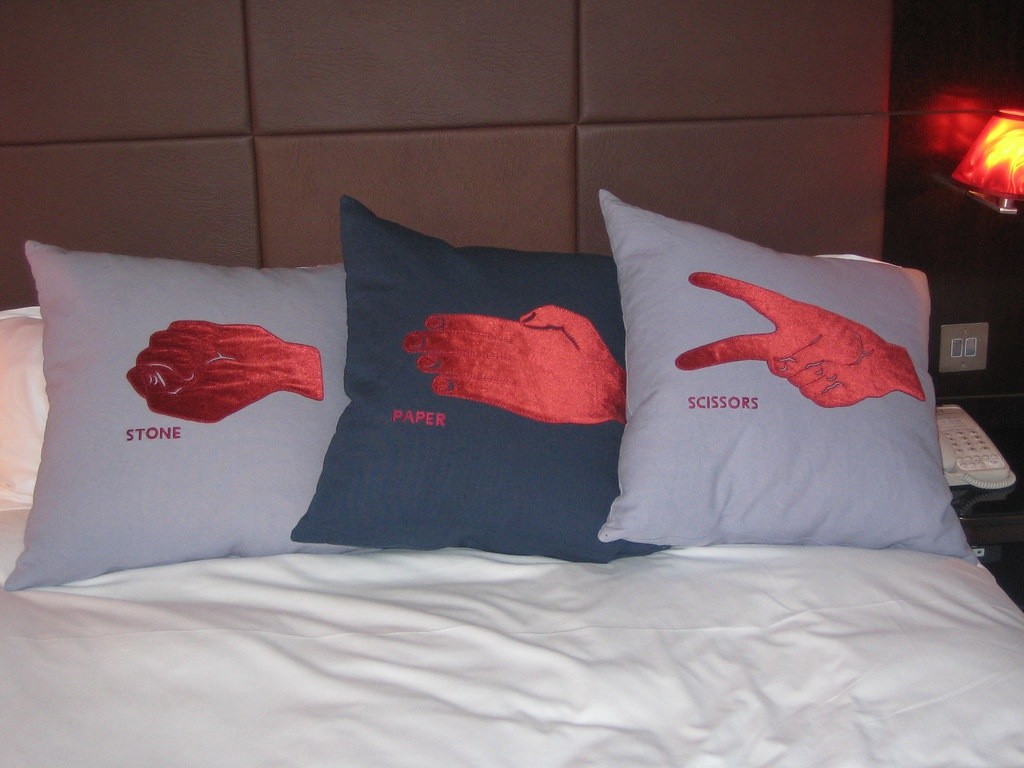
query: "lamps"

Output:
[931,107,1024,215]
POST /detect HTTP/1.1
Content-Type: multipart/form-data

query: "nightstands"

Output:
[934,395,1024,609]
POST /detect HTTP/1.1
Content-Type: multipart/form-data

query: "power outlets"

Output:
[970,545,1001,564]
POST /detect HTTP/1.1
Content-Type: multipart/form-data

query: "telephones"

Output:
[935,402,1010,487]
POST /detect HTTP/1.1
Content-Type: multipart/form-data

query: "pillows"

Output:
[596,188,983,568]
[4,239,382,592]
[292,193,672,565]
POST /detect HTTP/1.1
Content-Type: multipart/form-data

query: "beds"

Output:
[0,1,1023,768]
